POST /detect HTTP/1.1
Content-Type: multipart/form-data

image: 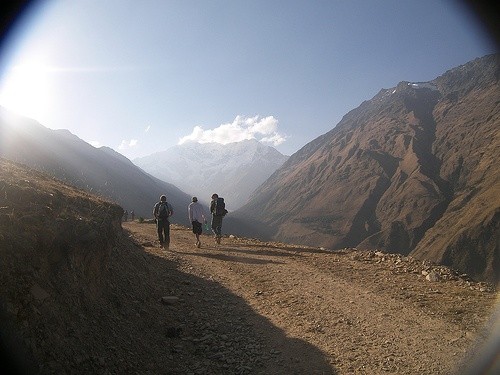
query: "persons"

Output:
[131,208,135,221]
[187,196,206,248]
[152,195,175,249]
[209,193,227,244]
[124,209,129,222]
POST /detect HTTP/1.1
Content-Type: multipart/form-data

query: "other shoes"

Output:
[165,246,169,250]
[215,242,220,245]
[196,242,200,247]
[160,242,164,248]
[214,235,220,239]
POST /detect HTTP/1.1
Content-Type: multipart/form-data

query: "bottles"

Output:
[205,223,209,231]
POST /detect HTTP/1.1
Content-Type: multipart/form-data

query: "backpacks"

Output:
[214,198,225,217]
[158,202,168,218]
